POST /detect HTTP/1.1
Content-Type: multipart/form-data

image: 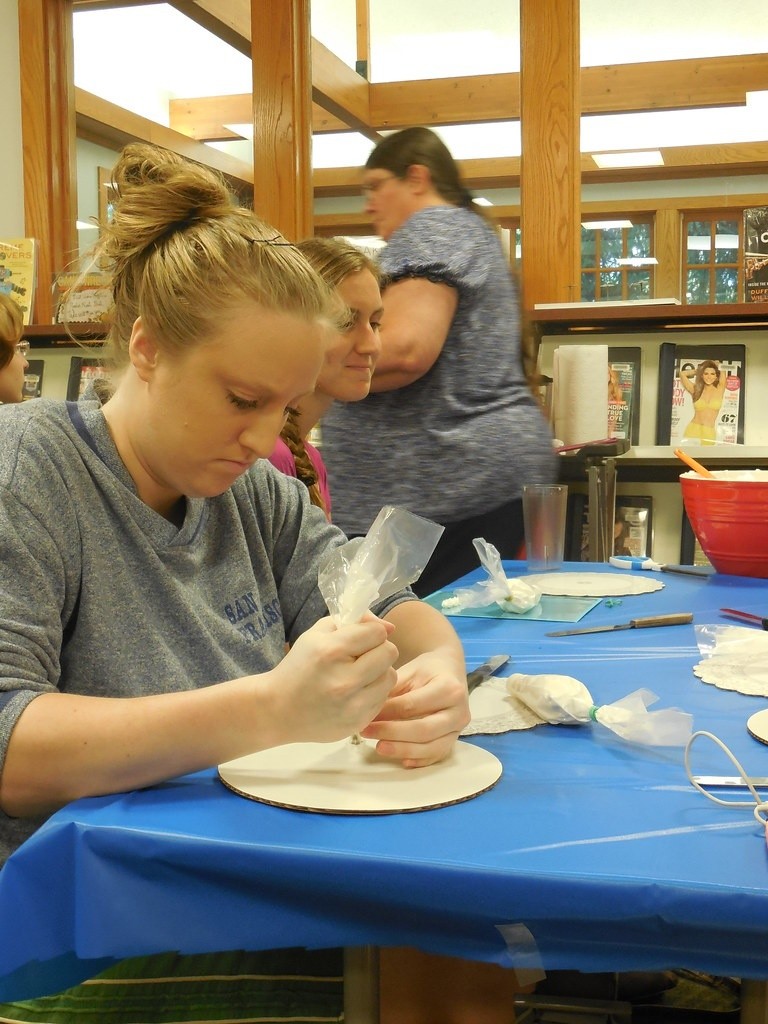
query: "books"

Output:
[0,238,38,325]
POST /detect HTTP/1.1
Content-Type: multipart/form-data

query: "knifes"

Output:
[544,613,691,637]
[718,609,768,631]
[467,653,510,693]
[691,776,768,787]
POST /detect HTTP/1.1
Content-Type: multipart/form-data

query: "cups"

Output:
[521,483,567,572]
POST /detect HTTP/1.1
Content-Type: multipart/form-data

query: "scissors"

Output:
[609,555,710,577]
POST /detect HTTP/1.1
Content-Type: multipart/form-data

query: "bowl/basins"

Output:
[679,470,768,578]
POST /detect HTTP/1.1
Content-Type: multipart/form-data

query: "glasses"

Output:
[363,172,404,197]
[15,340,30,355]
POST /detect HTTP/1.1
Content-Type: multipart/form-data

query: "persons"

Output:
[319,128,559,602]
[268,238,385,524]
[0,292,30,403]
[0,143,470,871]
[608,366,623,440]
[681,361,730,447]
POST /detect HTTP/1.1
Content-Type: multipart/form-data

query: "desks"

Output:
[1,557,767,1024]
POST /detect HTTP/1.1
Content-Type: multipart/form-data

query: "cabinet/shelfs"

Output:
[19,301,768,566]
